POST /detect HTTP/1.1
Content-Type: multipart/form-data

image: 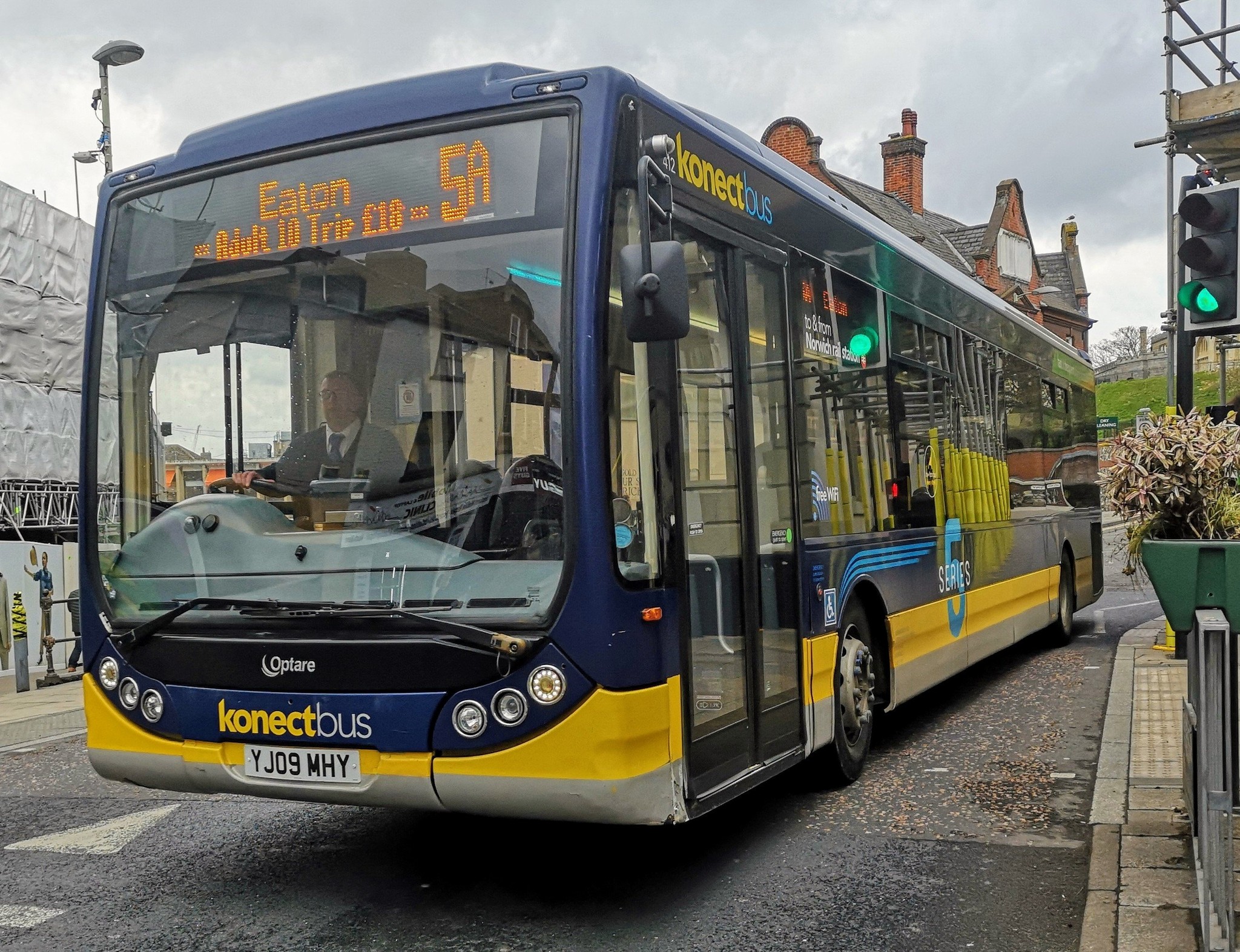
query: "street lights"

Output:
[93,42,147,176]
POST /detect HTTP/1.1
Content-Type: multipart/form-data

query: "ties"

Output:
[329,433,345,462]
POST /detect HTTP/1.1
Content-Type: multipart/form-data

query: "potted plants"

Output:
[1093,404,1240,631]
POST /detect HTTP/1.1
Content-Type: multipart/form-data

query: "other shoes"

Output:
[37,655,43,665]
[68,666,76,672]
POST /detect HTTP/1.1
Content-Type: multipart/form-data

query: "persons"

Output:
[67,589,82,673]
[232,371,406,518]
[0,573,12,670]
[24,551,54,666]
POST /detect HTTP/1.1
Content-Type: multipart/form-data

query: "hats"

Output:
[30,546,38,567]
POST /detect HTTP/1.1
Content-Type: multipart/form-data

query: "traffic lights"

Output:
[1177,179,1240,332]
[821,245,889,373]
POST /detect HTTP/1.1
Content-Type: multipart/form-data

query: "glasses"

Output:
[319,387,351,400]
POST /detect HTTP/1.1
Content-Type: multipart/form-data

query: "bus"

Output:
[75,60,1106,827]
